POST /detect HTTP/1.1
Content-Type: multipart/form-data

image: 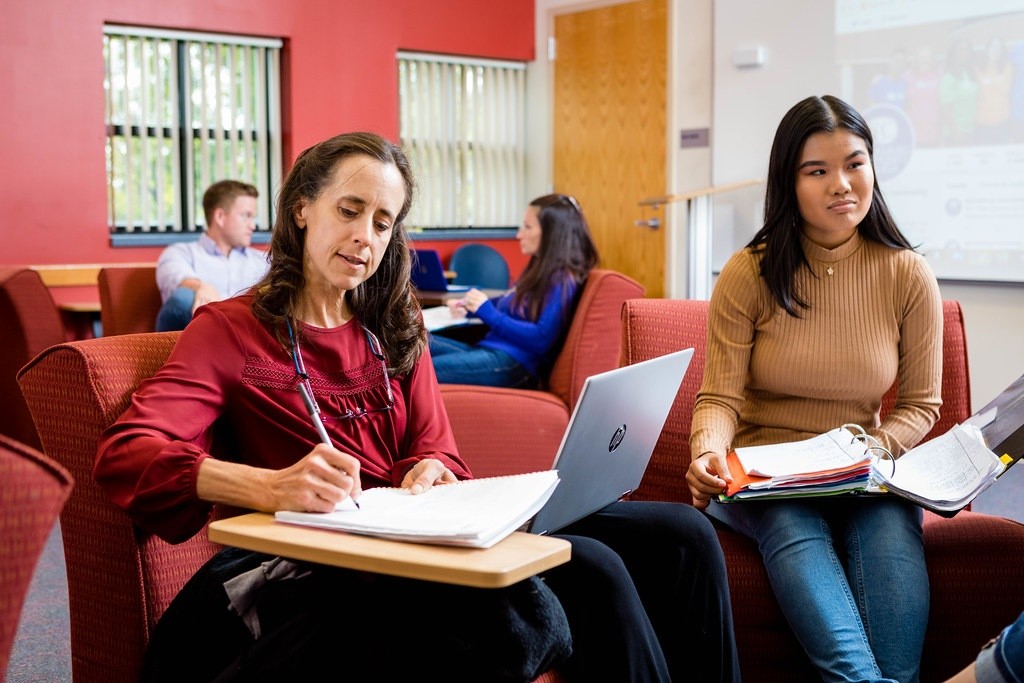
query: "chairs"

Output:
[448,243,510,290]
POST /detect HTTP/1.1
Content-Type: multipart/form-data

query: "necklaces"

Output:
[806,243,861,275]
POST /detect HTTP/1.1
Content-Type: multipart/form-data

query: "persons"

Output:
[684,92,943,683]
[425,192,600,388]
[152,179,278,333]
[92,131,746,683]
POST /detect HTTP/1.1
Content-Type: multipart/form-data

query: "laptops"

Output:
[408,249,480,292]
[517,348,695,536]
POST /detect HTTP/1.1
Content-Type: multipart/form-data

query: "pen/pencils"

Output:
[298,382,361,509]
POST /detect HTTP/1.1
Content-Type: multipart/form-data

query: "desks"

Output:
[416,288,503,305]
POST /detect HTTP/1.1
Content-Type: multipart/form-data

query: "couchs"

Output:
[615,298,1024,682]
[14,327,251,682]
[436,270,644,477]
[98,266,165,336]
[0,267,68,456]
